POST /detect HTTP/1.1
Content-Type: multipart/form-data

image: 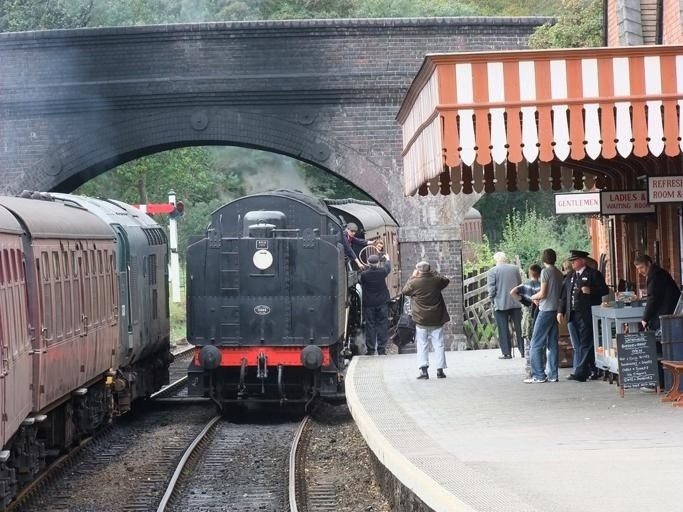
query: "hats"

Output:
[415,262,429,272]
[367,255,377,263]
[347,222,357,231]
[567,250,588,261]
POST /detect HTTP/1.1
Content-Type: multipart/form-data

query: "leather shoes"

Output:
[498,355,510,359]
[570,374,585,382]
[588,374,596,380]
[379,352,386,355]
[365,352,373,355]
[437,373,446,378]
[416,373,428,379]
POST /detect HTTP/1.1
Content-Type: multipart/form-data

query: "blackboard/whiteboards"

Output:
[618,332,660,387]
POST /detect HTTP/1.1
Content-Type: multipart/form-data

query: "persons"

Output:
[633,253,680,388]
[485,251,527,360]
[341,221,376,272]
[556,249,608,382]
[401,260,450,380]
[510,264,547,383]
[521,248,563,383]
[356,251,393,355]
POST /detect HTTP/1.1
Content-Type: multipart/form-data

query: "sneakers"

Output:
[522,377,545,383]
[565,373,574,380]
[546,378,556,382]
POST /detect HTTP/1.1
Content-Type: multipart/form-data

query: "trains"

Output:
[187,188,481,424]
[1,188,172,512]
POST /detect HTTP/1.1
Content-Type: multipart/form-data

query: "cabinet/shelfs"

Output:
[590,303,646,383]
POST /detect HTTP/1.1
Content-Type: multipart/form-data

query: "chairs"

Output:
[659,353,683,407]
